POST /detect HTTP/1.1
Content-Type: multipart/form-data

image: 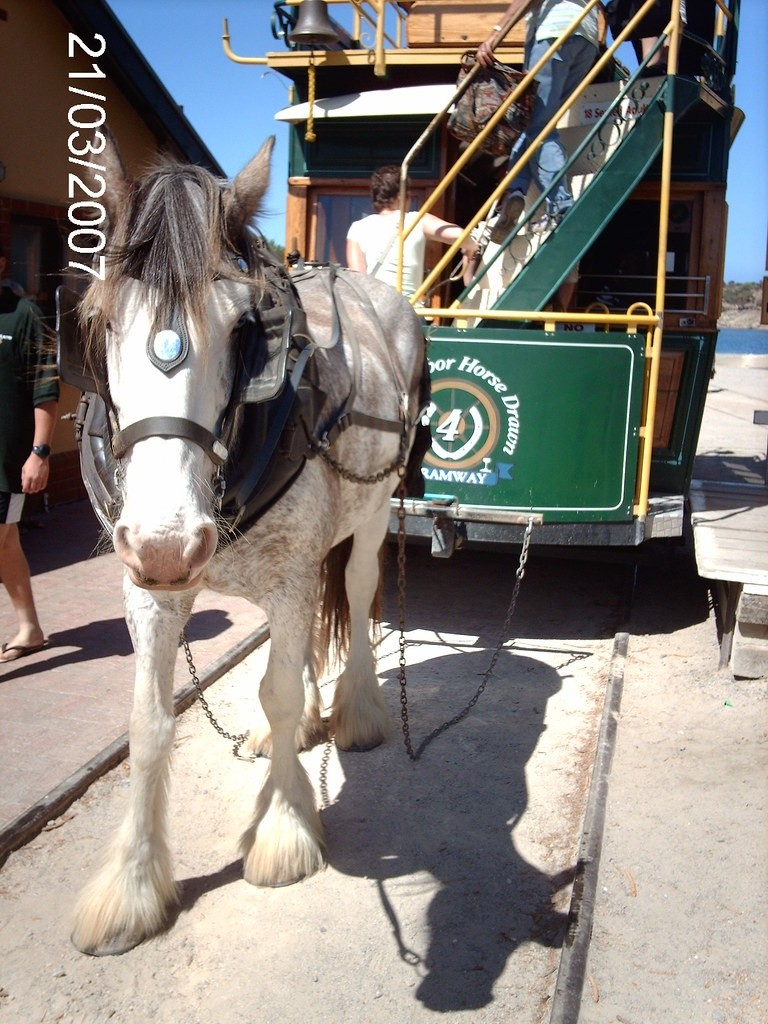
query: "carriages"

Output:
[74,0,746,958]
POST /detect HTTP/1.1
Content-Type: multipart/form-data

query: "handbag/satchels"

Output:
[447,51,542,156]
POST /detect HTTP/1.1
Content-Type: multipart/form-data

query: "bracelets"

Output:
[492,24,502,32]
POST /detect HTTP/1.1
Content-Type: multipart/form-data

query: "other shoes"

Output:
[544,210,566,231]
[489,190,525,244]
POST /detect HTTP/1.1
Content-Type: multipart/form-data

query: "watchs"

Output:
[32,444,51,459]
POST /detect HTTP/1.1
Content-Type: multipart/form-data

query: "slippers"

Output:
[0,638,52,662]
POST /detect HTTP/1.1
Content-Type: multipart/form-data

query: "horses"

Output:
[65,133,430,959]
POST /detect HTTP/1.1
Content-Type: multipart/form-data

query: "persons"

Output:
[475,0,607,245]
[1,252,62,662]
[345,165,476,330]
